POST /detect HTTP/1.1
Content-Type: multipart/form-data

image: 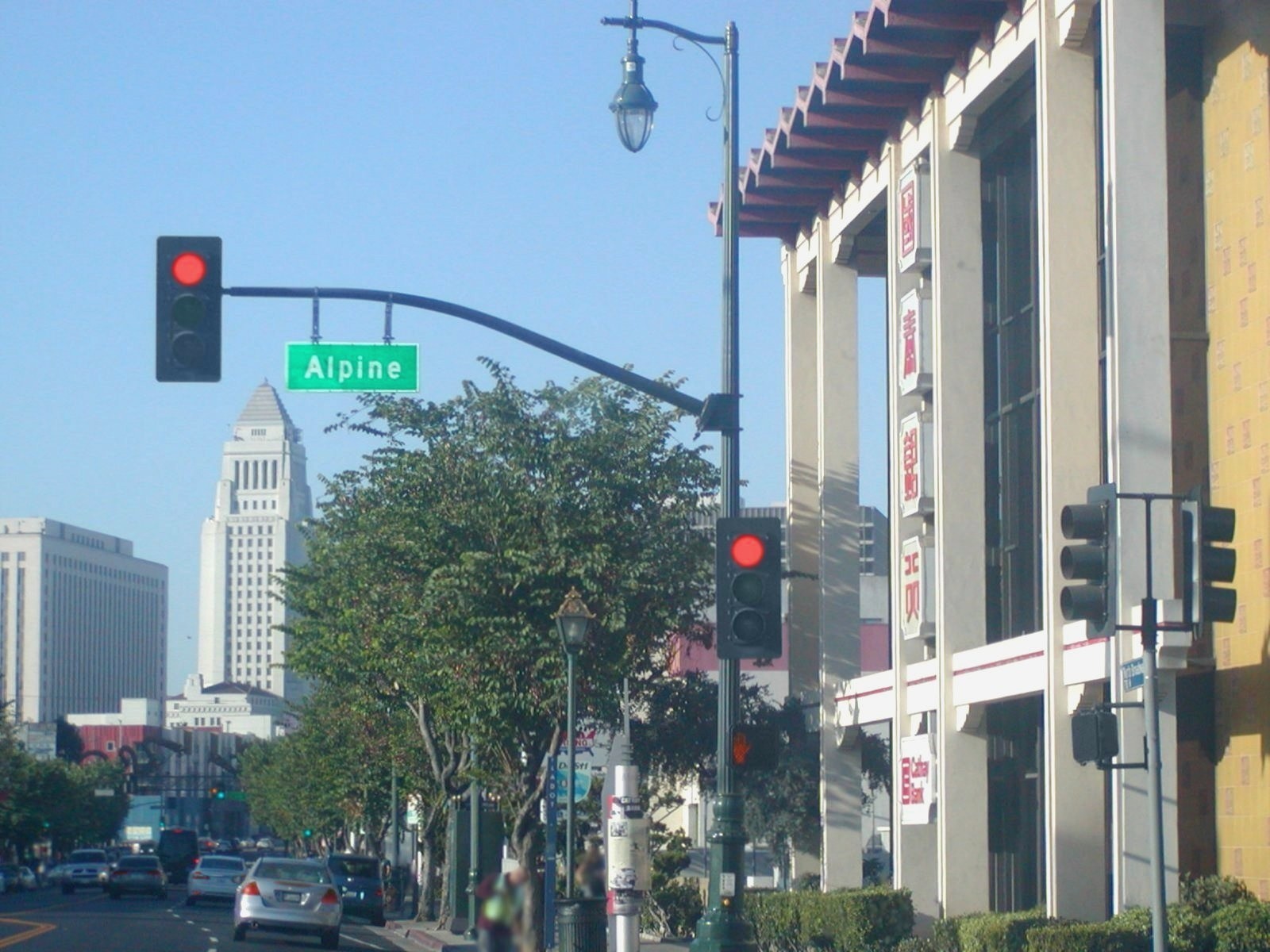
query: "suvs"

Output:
[64,849,111,892]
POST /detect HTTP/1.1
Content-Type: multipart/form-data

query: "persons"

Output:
[477,868,532,952]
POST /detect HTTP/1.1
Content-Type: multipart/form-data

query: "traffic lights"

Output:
[1179,486,1238,637]
[304,828,313,836]
[211,782,226,800]
[1059,483,1117,641]
[1071,708,1118,767]
[716,517,784,661]
[153,235,224,386]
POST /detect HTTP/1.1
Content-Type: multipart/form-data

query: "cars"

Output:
[0,863,64,896]
[111,855,168,901]
[319,854,385,926]
[233,855,342,948]
[187,855,247,905]
[196,835,278,855]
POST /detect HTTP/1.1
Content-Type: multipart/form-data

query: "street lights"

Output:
[374,670,404,913]
[551,586,600,952]
[602,0,751,950]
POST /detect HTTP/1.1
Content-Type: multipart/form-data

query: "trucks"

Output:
[156,827,199,882]
